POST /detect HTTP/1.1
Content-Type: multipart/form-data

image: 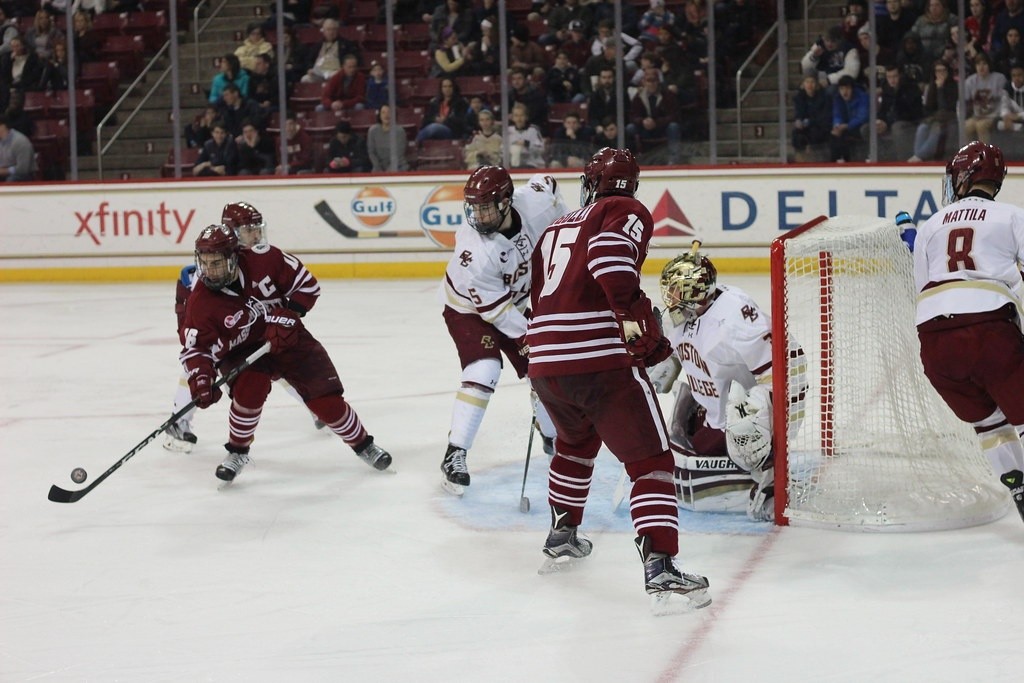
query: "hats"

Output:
[644,70,659,82]
[509,27,531,44]
[569,19,585,34]
[858,20,872,38]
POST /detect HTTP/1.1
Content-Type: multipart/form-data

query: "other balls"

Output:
[70,467,87,483]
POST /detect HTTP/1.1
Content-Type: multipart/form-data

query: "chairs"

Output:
[0,0,779,171]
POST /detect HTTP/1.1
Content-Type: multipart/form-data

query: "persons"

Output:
[529,147,712,614]
[180,225,397,492]
[439,165,556,498]
[791,0,1024,165]
[658,252,807,519]
[0,0,112,186]
[162,205,261,454]
[185,0,410,182]
[397,0,746,175]
[912,139,1024,524]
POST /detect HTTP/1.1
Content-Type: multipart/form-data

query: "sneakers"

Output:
[439,432,470,494]
[352,434,394,474]
[538,506,593,575]
[215,435,255,491]
[163,404,197,455]
[312,414,331,434]
[1000,469,1024,523]
[534,421,556,463]
[634,534,711,616]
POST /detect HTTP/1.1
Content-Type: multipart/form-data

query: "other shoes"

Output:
[906,155,921,162]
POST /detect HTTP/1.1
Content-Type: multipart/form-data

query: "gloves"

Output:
[261,308,301,355]
[632,337,675,369]
[187,366,222,409]
[515,332,531,361]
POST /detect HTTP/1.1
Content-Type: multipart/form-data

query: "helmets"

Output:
[219,201,262,248]
[194,225,240,289]
[659,252,717,327]
[947,140,1008,200]
[462,164,514,235]
[579,147,641,205]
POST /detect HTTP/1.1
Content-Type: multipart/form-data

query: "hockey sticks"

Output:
[629,306,664,346]
[46,340,271,503]
[519,417,540,513]
[313,199,426,241]
